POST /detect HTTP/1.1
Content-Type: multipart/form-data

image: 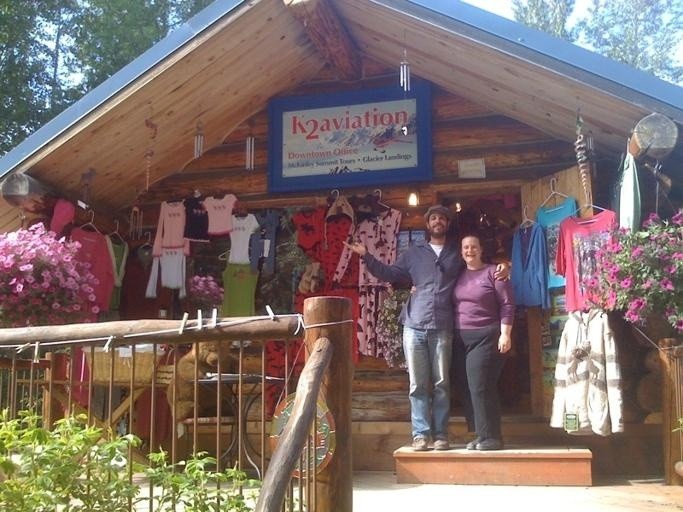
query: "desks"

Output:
[195,373,291,490]
[17,379,169,472]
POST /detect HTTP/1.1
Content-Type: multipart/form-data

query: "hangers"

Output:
[81,189,392,262]
[519,136,632,230]
[570,287,601,317]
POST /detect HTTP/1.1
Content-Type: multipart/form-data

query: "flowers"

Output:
[0,219,99,327]
[187,275,225,300]
[582,210,683,335]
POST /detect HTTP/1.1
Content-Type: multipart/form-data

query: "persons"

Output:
[341,203,510,452]
[409,235,516,451]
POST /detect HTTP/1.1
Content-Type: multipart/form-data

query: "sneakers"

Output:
[412,438,428,451]
[466,437,504,450]
[434,439,448,450]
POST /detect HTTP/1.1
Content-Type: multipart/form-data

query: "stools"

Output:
[180,416,237,471]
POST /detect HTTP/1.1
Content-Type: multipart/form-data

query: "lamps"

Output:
[403,185,426,214]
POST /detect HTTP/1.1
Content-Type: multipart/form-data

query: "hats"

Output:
[424,205,452,225]
